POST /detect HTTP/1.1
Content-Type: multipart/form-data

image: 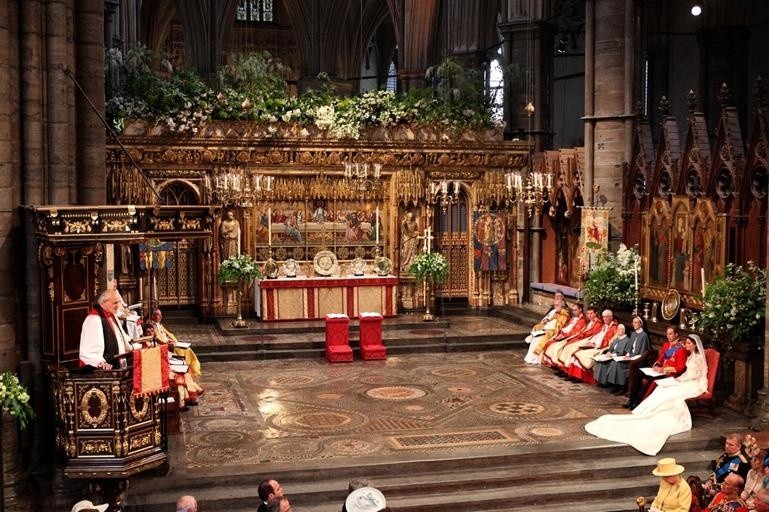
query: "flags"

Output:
[471,210,508,270]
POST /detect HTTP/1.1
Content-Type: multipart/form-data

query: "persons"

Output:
[340,478,391,512]
[266,496,292,512]
[256,479,283,512]
[220,211,241,263]
[79,279,204,410]
[260,204,381,244]
[399,212,420,272]
[176,495,198,512]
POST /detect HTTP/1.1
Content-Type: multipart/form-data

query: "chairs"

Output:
[550,309,719,417]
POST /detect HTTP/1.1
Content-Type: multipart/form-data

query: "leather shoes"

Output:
[623,400,641,410]
[555,371,581,383]
[610,386,626,395]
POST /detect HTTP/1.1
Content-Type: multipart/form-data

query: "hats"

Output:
[652,457,685,477]
[71,500,110,512]
[345,487,386,512]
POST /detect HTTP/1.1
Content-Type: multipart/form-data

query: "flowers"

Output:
[692,260,768,338]
[0,371,38,432]
[579,242,643,308]
[218,255,261,286]
[407,252,449,284]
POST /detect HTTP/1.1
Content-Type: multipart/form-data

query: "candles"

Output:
[430,182,459,195]
[375,206,380,245]
[268,207,272,246]
[634,259,638,289]
[154,276,157,301]
[505,172,554,190]
[344,163,381,179]
[701,267,706,297]
[423,225,432,254]
[237,227,241,256]
[203,172,270,193]
[139,277,143,301]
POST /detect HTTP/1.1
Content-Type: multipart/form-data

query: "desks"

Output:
[253,272,399,320]
[718,430,769,460]
